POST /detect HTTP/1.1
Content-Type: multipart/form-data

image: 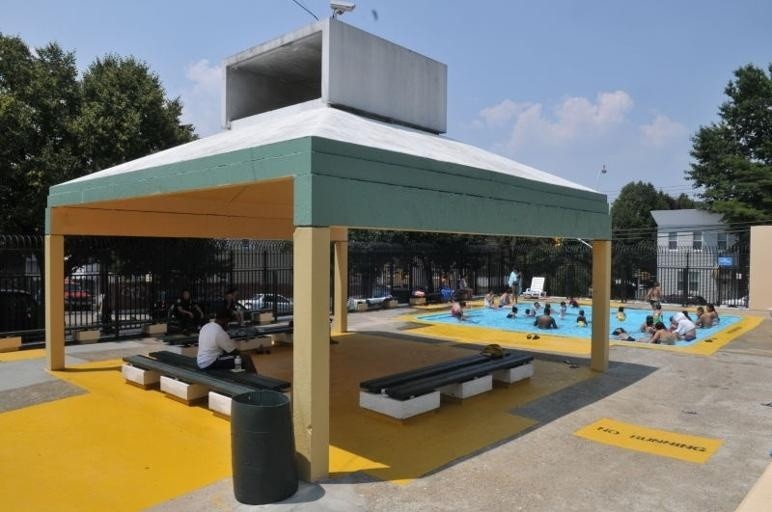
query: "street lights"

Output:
[595,165,610,193]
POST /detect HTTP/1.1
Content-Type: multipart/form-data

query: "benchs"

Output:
[162,323,293,354]
[121,351,292,418]
[349,288,471,311]
[357,346,533,421]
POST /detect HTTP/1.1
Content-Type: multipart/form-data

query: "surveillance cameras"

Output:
[329,0,356,14]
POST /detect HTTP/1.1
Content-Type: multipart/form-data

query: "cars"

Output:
[663,292,706,304]
[348,290,395,310]
[427,287,456,302]
[235,293,292,315]
[386,283,409,303]
[720,295,748,307]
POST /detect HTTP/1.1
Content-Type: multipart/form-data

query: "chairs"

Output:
[524,275,550,300]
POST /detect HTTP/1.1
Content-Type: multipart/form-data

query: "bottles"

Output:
[234,355,241,371]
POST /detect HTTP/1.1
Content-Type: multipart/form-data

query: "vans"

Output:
[2,288,68,341]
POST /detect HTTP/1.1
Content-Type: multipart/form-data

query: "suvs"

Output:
[64,277,94,311]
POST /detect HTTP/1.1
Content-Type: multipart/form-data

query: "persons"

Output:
[329,318,339,344]
[175,289,247,336]
[197,309,257,373]
[441,267,720,345]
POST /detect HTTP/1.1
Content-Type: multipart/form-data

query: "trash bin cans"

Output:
[231,390,298,505]
[441,288,452,304]
